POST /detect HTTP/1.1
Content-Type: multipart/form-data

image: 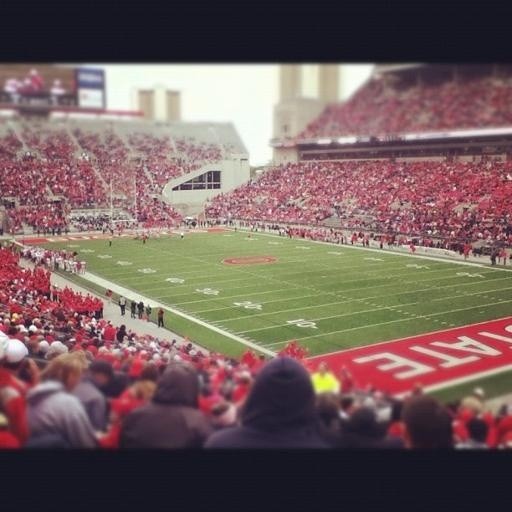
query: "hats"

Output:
[0,331,28,364]
[40,340,68,359]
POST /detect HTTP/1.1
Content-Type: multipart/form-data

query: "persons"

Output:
[5,68,66,103]
